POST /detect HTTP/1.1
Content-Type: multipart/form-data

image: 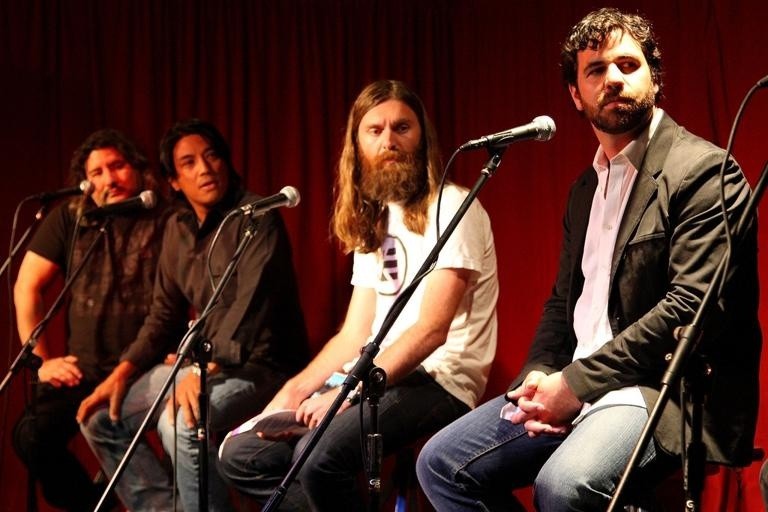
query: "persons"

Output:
[12,127,174,512]
[213,79,500,512]
[75,117,312,512]
[415,6,763,512]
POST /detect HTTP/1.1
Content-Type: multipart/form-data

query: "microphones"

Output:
[224,185,303,226]
[459,115,558,156]
[81,190,161,224]
[27,178,95,204]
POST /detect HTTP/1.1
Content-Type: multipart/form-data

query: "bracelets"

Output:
[341,384,361,400]
[189,364,213,382]
